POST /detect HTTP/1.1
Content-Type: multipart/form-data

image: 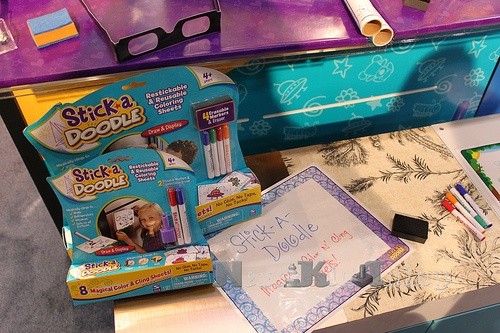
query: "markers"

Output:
[167,188,192,247]
[442,182,493,242]
[202,123,235,181]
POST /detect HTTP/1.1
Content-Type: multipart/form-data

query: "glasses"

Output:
[78,0,222,64]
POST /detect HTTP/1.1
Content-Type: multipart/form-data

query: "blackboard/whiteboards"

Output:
[202,164,411,333]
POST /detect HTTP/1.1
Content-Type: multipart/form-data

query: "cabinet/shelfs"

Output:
[110,112,500,332]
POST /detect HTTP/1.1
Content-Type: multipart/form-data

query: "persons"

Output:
[115,202,176,255]
[147,139,199,166]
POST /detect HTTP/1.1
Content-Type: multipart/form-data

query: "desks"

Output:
[0,0,500,244]
